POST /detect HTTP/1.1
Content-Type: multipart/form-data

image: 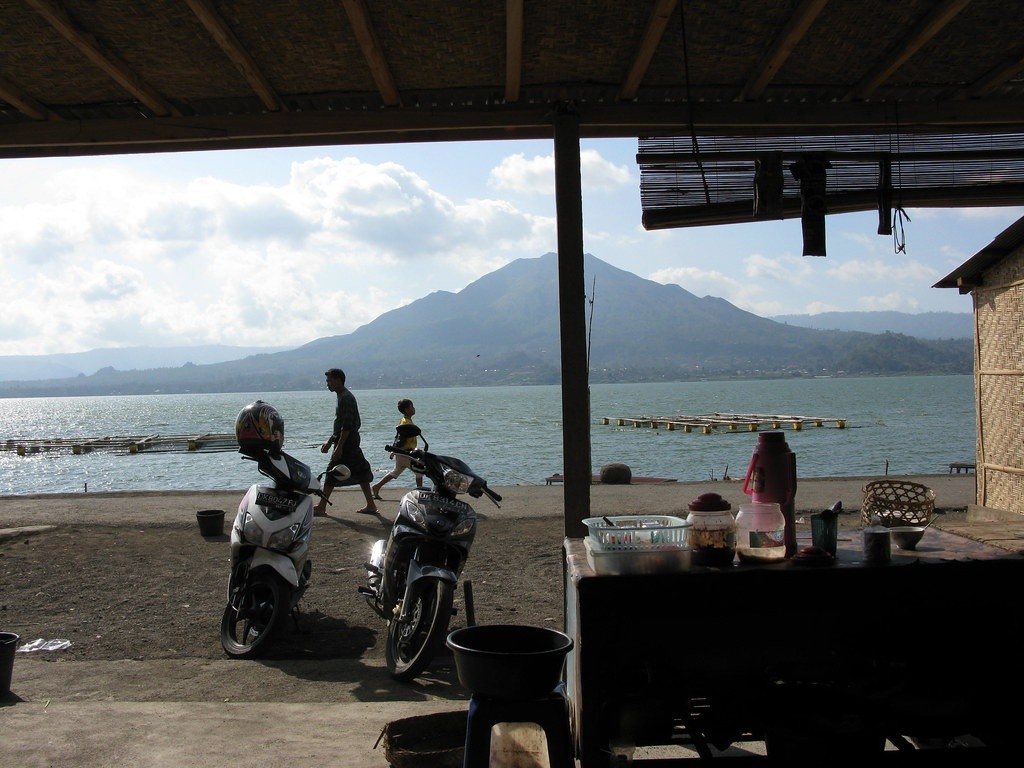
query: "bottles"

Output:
[687,493,735,566]
[736,503,786,564]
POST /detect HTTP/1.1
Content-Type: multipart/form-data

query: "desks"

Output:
[564,527,1024,768]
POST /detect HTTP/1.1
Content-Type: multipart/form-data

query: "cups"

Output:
[810,514,837,557]
[860,527,892,562]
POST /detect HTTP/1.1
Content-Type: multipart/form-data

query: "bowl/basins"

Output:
[889,526,926,550]
[447,622,574,703]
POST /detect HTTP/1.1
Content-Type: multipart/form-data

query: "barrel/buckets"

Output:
[195,509,226,537]
[0,631,20,698]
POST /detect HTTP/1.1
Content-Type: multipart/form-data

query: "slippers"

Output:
[356,506,379,514]
[313,505,326,515]
[372,485,382,499]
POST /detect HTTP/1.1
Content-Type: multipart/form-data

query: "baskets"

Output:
[861,481,937,531]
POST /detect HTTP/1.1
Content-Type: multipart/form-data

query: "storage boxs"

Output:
[583,537,691,573]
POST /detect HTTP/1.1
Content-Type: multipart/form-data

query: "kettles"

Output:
[742,432,798,558]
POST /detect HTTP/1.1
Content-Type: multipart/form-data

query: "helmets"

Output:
[235,400,284,448]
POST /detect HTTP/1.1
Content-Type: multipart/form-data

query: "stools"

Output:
[463,681,575,768]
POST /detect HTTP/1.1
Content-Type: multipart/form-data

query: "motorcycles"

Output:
[361,425,502,681]
[219,446,353,659]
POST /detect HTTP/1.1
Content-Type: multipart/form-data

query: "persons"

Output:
[313,368,378,515]
[372,399,429,500]
